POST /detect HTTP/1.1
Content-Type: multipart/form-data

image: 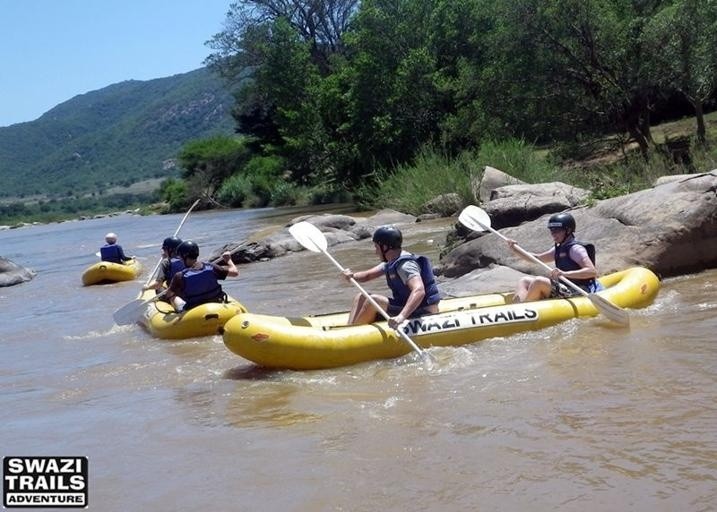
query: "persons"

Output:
[142,234,184,302]
[100,233,136,266]
[341,225,440,330]
[507,210,600,304]
[163,239,239,316]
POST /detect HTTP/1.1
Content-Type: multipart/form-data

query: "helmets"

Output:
[104,233,118,244]
[175,240,199,258]
[161,236,182,249]
[546,212,575,234]
[372,225,402,249]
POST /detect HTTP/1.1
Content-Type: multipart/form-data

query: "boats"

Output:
[80,257,144,285]
[219,267,662,369]
[135,277,247,339]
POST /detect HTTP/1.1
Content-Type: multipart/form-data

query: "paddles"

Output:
[113,225,280,327]
[458,205,631,328]
[95,251,146,261]
[289,221,422,352]
[139,198,200,298]
